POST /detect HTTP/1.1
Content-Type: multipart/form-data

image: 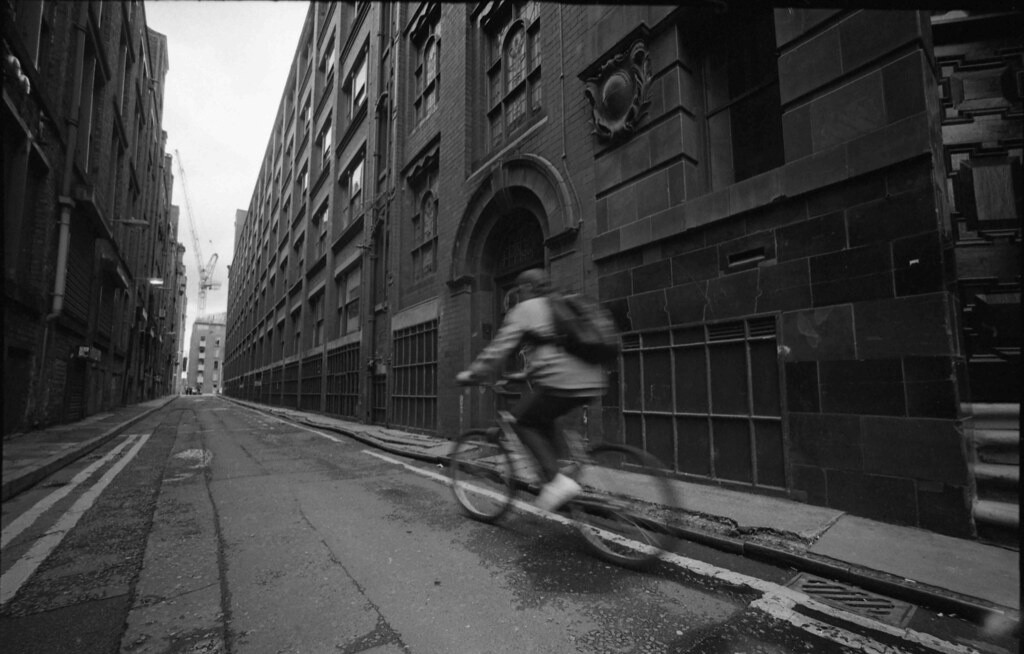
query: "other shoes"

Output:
[534,473,581,514]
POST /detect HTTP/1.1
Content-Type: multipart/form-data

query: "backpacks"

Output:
[511,288,623,364]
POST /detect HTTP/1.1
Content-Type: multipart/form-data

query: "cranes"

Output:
[175,149,221,322]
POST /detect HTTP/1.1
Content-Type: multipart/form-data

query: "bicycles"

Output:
[450,381,679,569]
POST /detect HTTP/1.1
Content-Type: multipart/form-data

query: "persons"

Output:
[184,384,192,395]
[196,383,201,395]
[456,269,611,511]
